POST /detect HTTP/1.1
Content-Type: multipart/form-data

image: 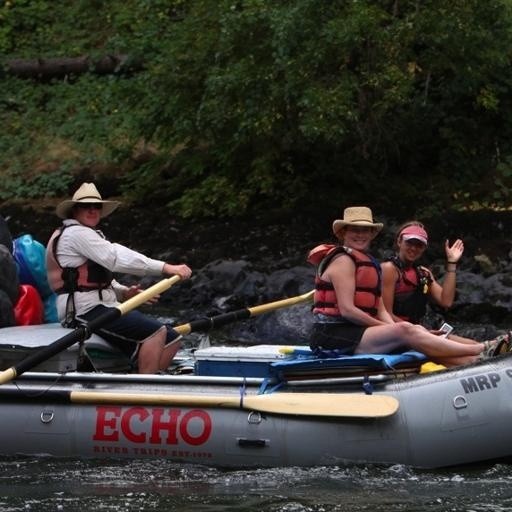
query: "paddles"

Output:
[4,391,400,418]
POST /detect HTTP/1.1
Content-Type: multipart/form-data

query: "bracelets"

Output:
[444,266,459,273]
[447,262,458,264]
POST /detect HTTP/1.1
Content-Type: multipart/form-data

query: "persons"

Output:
[380,222,481,368]
[311,206,512,365]
[46,183,192,374]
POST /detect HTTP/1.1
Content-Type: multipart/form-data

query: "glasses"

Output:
[343,227,371,234]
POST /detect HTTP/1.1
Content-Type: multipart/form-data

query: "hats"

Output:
[56,182,121,219]
[332,207,384,240]
[399,225,427,246]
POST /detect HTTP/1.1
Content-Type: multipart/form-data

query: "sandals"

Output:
[480,340,507,361]
[72,203,103,209]
[484,330,512,350]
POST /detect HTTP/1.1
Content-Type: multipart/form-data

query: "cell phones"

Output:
[437,321,455,340]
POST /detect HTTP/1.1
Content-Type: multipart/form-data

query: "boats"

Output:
[1,321,512,472]
[0,343,512,477]
